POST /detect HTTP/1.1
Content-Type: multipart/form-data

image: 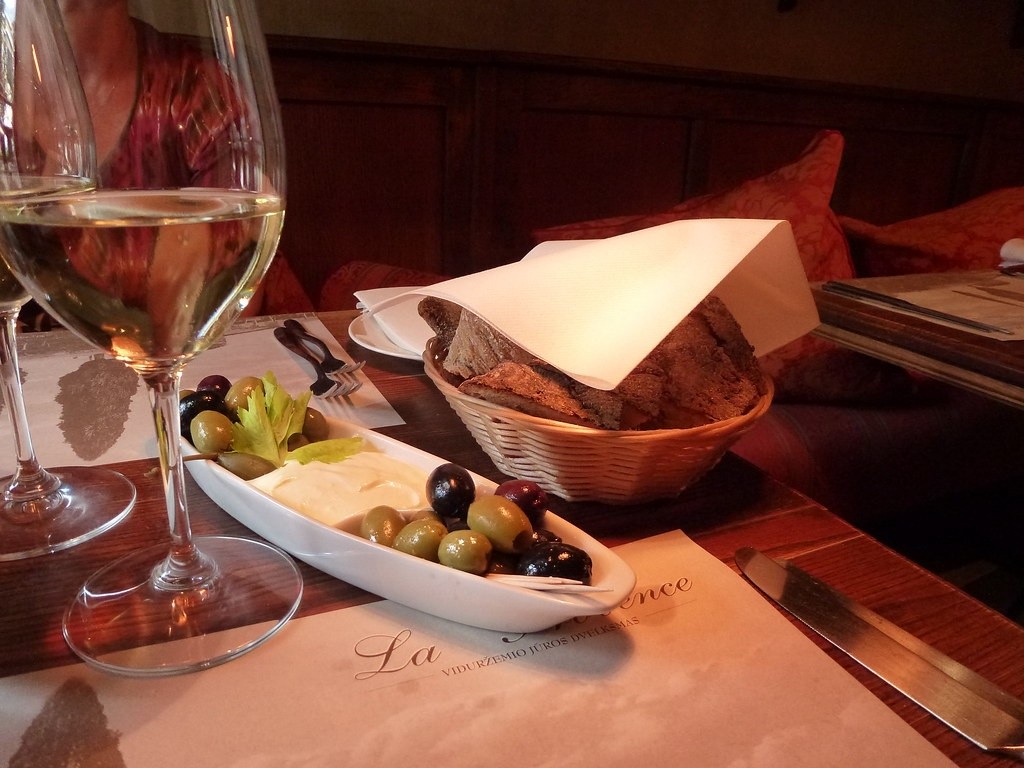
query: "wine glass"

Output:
[0,1,303,674]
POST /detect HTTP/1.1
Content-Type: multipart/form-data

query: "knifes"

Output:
[734,547,1024,765]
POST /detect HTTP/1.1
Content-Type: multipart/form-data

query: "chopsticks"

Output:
[822,281,1013,335]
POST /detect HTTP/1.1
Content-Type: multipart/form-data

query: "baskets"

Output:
[422,335,775,504]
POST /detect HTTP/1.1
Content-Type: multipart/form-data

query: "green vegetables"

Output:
[229,370,364,469]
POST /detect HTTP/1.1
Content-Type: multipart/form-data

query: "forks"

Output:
[274,327,363,399]
[284,319,366,375]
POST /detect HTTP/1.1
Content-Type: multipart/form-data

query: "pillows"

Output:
[530,128,909,404]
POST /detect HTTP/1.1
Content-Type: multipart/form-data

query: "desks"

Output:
[0,310,1024,768]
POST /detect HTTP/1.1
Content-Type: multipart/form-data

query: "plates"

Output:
[178,388,636,634]
[348,315,423,361]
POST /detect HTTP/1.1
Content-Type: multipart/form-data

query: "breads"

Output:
[418,296,764,428]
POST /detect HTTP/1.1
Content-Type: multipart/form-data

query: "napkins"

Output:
[351,219,824,391]
